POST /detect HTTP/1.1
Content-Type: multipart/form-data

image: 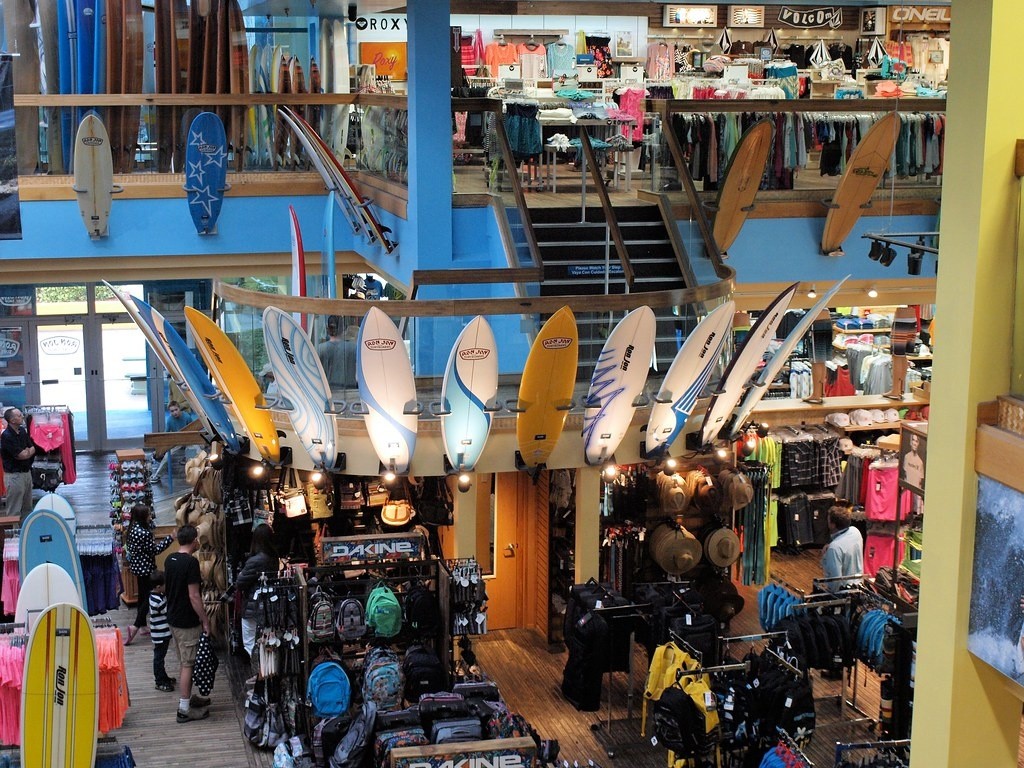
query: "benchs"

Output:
[545,140,637,194]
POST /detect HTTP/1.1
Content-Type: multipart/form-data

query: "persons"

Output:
[237,523,288,658]
[258,362,280,396]
[902,433,924,490]
[149,570,176,692]
[124,505,177,646]
[0,408,37,534]
[163,525,211,723]
[822,505,864,587]
[316,315,364,393]
[149,401,194,483]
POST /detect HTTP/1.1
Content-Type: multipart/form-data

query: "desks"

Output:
[533,114,660,192]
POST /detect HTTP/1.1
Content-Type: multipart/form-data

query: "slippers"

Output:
[123,625,134,646]
[140,627,151,636]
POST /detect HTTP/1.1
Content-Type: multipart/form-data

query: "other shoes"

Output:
[169,678,176,683]
[155,683,174,692]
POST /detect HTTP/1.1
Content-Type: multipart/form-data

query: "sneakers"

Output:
[176,707,209,723]
[190,694,210,707]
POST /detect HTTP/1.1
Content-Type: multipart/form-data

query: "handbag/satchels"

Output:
[243,676,303,749]
[192,632,220,696]
[276,467,307,518]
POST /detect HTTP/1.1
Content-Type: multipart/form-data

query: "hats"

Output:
[175,449,226,640]
[653,470,754,575]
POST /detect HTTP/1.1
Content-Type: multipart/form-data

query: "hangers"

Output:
[785,34,847,49]
[465,77,781,98]
[846,341,893,363]
[24,404,74,417]
[9,734,126,765]
[490,32,575,48]
[2,522,115,562]
[0,618,120,658]
[734,54,792,64]
[672,105,942,120]
[779,727,910,768]
[375,74,394,82]
[650,34,711,47]
[669,577,889,684]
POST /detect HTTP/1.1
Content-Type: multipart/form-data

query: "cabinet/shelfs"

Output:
[343,63,376,163]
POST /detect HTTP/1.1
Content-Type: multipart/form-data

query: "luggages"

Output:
[375,682,499,768]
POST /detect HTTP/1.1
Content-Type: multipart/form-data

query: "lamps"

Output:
[663,452,677,475]
[378,458,411,490]
[251,446,297,477]
[740,420,770,437]
[444,453,473,492]
[869,233,941,275]
[698,445,728,464]
[210,437,250,470]
[868,284,879,297]
[311,452,348,481]
[807,283,817,298]
[735,438,757,458]
[604,464,619,483]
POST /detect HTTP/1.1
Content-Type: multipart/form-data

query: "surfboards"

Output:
[276,103,394,254]
[645,300,737,461]
[821,109,903,255]
[583,304,657,468]
[286,189,337,335]
[12,562,81,634]
[72,114,114,239]
[33,493,78,540]
[352,304,422,478]
[710,116,775,255]
[1,0,350,174]
[727,272,853,440]
[185,110,228,234]
[696,279,802,450]
[16,508,90,614]
[98,276,282,465]
[515,303,579,471]
[440,314,499,473]
[260,304,340,471]
[17,602,100,768]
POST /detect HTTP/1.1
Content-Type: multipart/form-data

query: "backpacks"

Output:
[313,701,376,768]
[306,583,445,719]
[654,683,706,757]
[272,735,315,768]
